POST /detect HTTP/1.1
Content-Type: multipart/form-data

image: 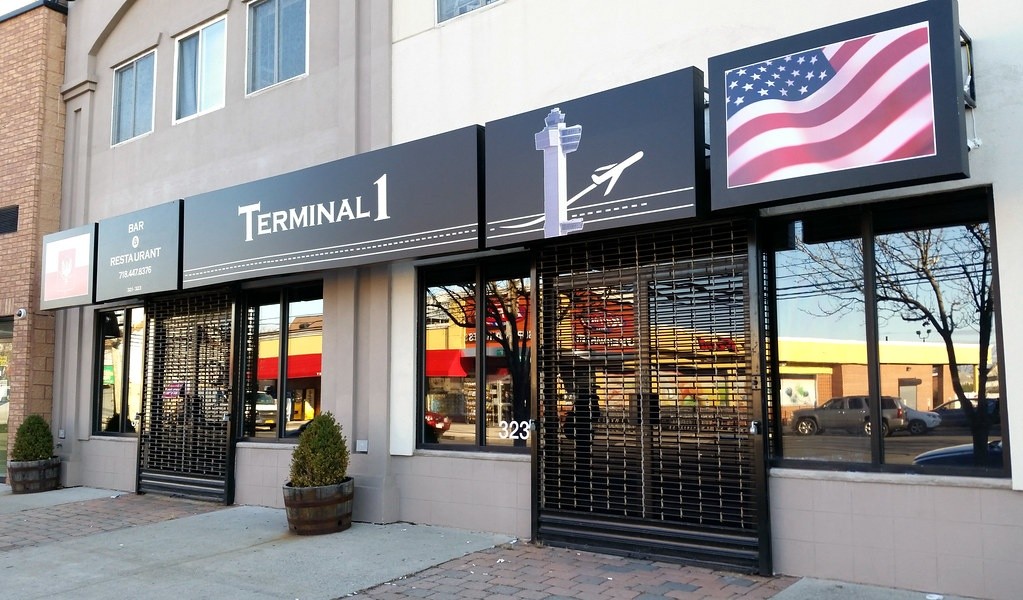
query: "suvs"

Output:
[792,395,904,438]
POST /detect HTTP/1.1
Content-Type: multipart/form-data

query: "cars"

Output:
[929,396,999,429]
[423,407,452,443]
[897,398,942,437]
[911,439,1003,468]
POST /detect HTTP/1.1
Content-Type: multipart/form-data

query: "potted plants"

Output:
[281,411,354,536]
[7,413,60,495]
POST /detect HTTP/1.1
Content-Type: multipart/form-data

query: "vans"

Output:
[244,391,276,430]
